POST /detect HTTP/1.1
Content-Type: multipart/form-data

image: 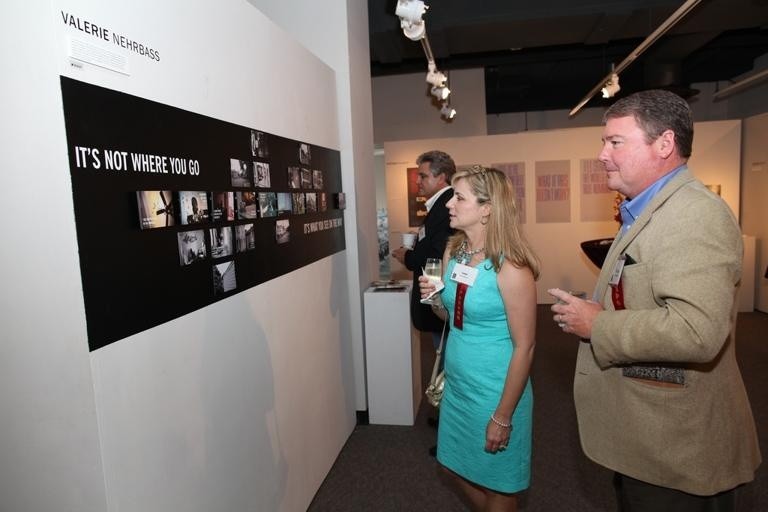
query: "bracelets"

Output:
[490,415,511,428]
[430,302,444,310]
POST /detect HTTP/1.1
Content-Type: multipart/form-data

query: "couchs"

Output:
[376,207,389,259]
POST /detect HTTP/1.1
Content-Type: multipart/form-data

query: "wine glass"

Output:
[422,256,444,307]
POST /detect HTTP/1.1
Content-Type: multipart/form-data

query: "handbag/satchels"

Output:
[425,369,445,410]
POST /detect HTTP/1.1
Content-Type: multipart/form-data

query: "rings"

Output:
[559,315,562,323]
[499,444,506,450]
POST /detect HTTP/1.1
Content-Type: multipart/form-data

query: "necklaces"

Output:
[456,240,488,264]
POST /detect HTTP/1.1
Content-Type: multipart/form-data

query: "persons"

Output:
[191,197,203,223]
[546,88,764,512]
[418,162,543,511]
[390,151,456,457]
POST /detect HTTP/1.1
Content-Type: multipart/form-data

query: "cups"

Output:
[557,292,587,329]
[403,234,416,248]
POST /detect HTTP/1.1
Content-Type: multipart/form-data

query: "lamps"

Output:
[395,0,456,121]
[600,73,621,99]
